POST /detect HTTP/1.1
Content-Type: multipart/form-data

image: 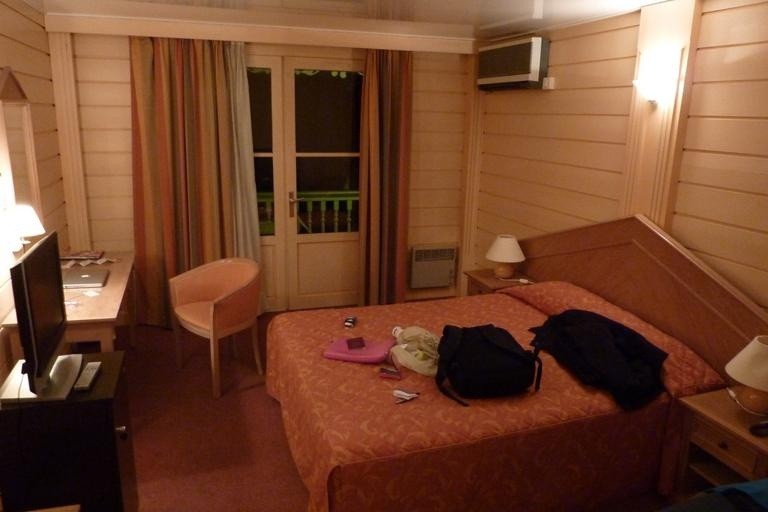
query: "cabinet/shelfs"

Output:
[0,352,137,512]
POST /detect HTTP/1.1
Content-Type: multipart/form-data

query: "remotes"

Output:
[73,362,102,391]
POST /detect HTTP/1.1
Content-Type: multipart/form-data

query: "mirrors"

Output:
[1,100,45,237]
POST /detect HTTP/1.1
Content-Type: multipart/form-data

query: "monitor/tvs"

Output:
[0,230,83,404]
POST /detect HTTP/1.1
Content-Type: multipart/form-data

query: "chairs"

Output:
[168,258,261,399]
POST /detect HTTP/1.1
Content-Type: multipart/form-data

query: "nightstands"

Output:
[465,268,535,295]
[679,386,768,496]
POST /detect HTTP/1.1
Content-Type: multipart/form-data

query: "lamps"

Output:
[6,202,45,253]
[726,334,768,416]
[487,235,526,279]
[632,79,670,109]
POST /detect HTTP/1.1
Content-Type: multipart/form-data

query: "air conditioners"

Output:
[477,37,550,90]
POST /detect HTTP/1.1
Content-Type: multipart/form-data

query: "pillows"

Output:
[497,280,725,396]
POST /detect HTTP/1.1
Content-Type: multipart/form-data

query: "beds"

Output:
[267,215,768,512]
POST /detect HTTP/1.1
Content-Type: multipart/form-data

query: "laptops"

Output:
[62,269,108,288]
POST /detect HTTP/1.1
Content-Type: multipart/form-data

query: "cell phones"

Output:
[347,337,366,350]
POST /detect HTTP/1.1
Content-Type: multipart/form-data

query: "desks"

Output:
[0,253,136,365]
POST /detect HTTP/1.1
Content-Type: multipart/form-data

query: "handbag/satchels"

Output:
[436,324,536,402]
